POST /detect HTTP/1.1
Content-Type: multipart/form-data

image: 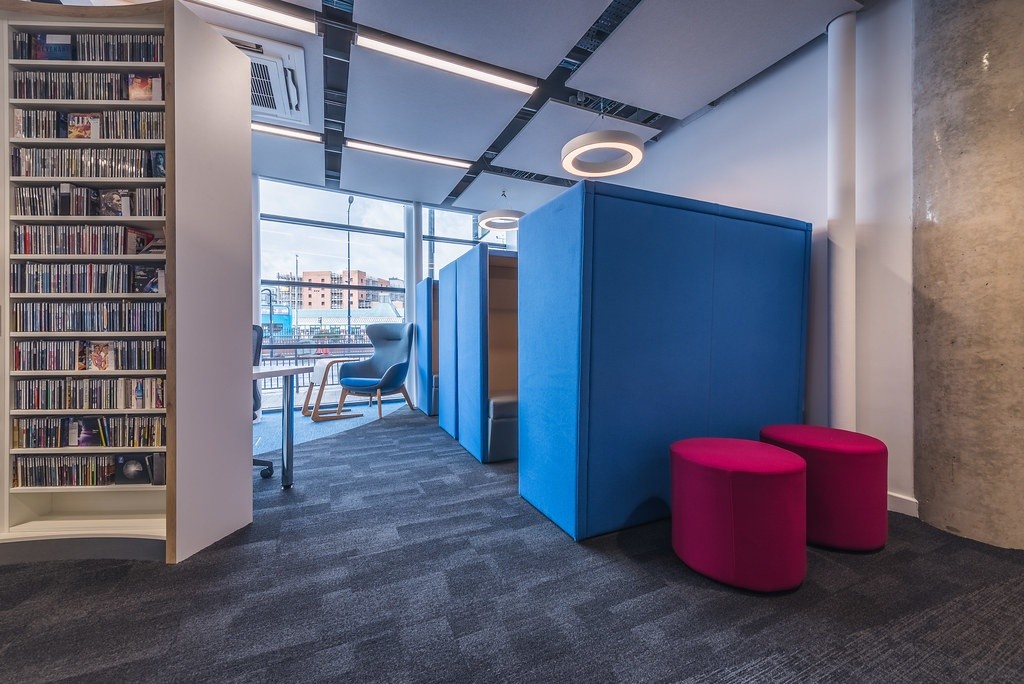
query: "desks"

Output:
[252,364,314,492]
[300,357,364,422]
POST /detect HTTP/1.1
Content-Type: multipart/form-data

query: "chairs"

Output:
[252,323,275,478]
[336,322,415,419]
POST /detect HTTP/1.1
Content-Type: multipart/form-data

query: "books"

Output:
[9,32,165,485]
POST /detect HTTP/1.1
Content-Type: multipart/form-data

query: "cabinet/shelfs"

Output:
[0,0,255,566]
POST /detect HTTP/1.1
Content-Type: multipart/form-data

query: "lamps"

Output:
[477,187,525,231]
[562,110,644,179]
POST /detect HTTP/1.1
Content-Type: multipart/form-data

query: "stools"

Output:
[671,436,806,592]
[759,423,888,549]
